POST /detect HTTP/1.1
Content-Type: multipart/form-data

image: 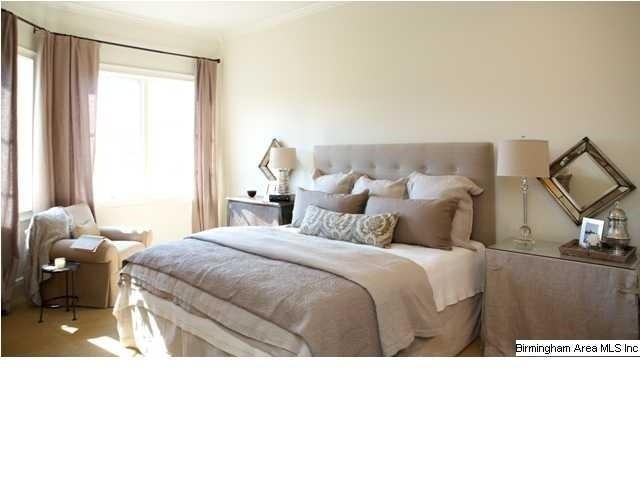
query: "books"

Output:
[69,233,105,255]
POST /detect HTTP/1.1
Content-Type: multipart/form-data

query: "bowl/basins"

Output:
[247,190,257,198]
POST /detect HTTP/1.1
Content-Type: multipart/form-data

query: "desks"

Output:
[36,264,79,322]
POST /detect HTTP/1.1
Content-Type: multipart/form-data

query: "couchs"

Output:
[30,202,153,309]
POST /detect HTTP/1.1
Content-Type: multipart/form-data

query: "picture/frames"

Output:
[577,216,605,249]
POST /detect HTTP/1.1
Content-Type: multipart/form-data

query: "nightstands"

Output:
[225,196,292,227]
[478,236,639,355]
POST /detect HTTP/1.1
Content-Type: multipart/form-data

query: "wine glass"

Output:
[606,201,631,257]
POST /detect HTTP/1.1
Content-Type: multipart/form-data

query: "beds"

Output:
[113,139,497,356]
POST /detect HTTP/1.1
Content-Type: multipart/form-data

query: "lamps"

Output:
[268,145,297,195]
[496,135,553,249]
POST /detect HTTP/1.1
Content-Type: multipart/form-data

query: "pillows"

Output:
[72,216,101,238]
[285,168,484,256]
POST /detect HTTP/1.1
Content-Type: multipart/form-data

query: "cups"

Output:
[54,257,66,268]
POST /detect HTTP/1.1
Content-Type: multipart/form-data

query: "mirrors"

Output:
[534,135,636,226]
[256,137,294,184]
